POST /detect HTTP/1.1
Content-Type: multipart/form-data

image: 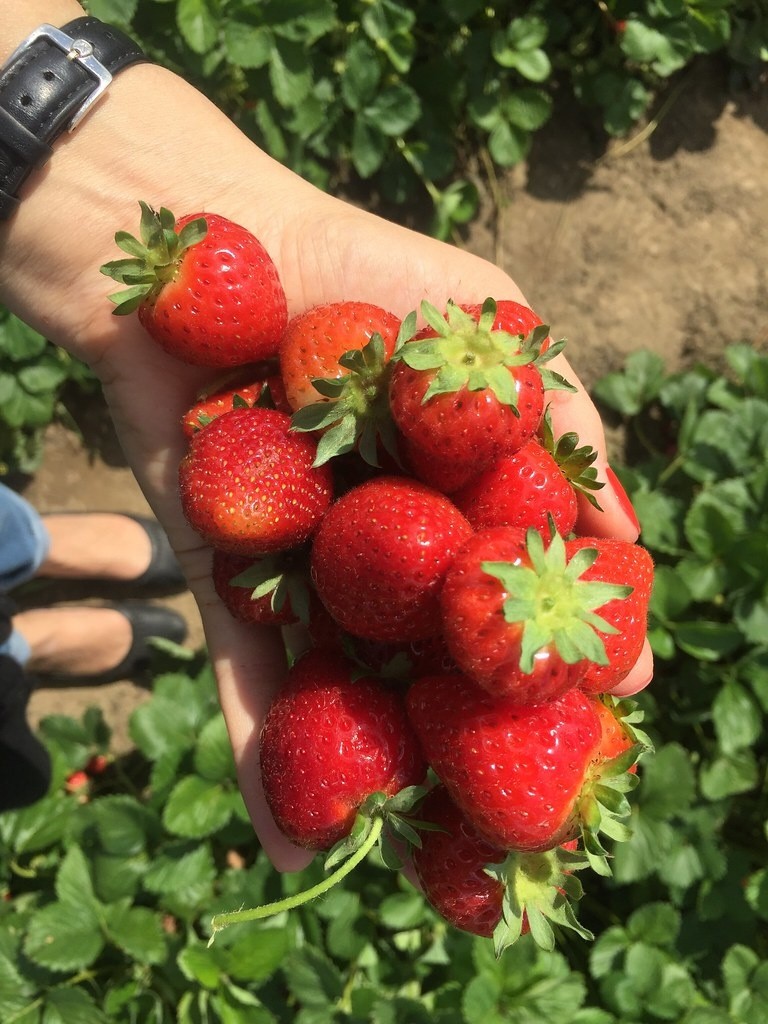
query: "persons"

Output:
[0,0,656,870]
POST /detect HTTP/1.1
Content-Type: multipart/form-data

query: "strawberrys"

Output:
[65,753,116,791]
[98,199,657,961]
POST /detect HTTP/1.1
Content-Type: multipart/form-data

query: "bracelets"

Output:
[0,16,147,218]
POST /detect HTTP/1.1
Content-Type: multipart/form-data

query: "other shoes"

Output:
[21,606,188,687]
[29,509,187,594]
